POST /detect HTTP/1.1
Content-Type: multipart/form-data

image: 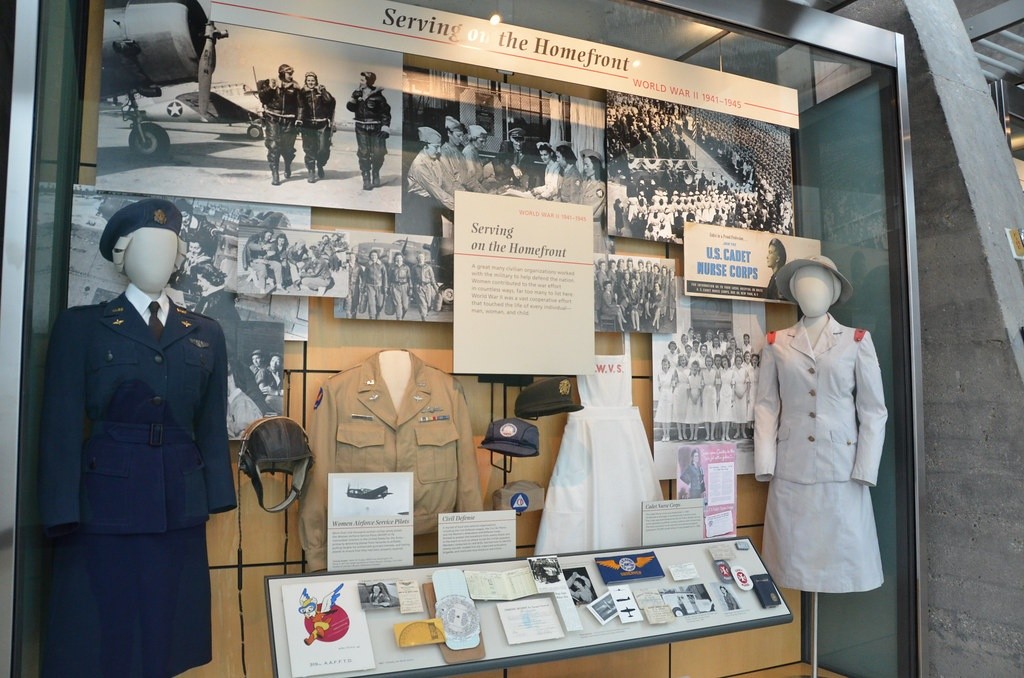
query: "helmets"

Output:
[241,416,313,513]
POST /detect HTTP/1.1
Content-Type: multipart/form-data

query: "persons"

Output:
[753,251,889,594]
[720,584,740,611]
[369,583,393,607]
[529,558,595,603]
[165,64,793,499]
[33,194,239,674]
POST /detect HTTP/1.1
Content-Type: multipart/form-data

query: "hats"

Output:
[361,71,376,85]
[580,149,602,162]
[99,199,180,261]
[478,418,539,457]
[769,238,786,262]
[515,377,583,419]
[776,255,853,308]
[468,125,487,138]
[305,72,318,77]
[279,64,294,74]
[444,116,461,128]
[417,126,442,144]
[510,127,527,138]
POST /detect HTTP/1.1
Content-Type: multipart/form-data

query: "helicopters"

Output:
[103,0,271,163]
